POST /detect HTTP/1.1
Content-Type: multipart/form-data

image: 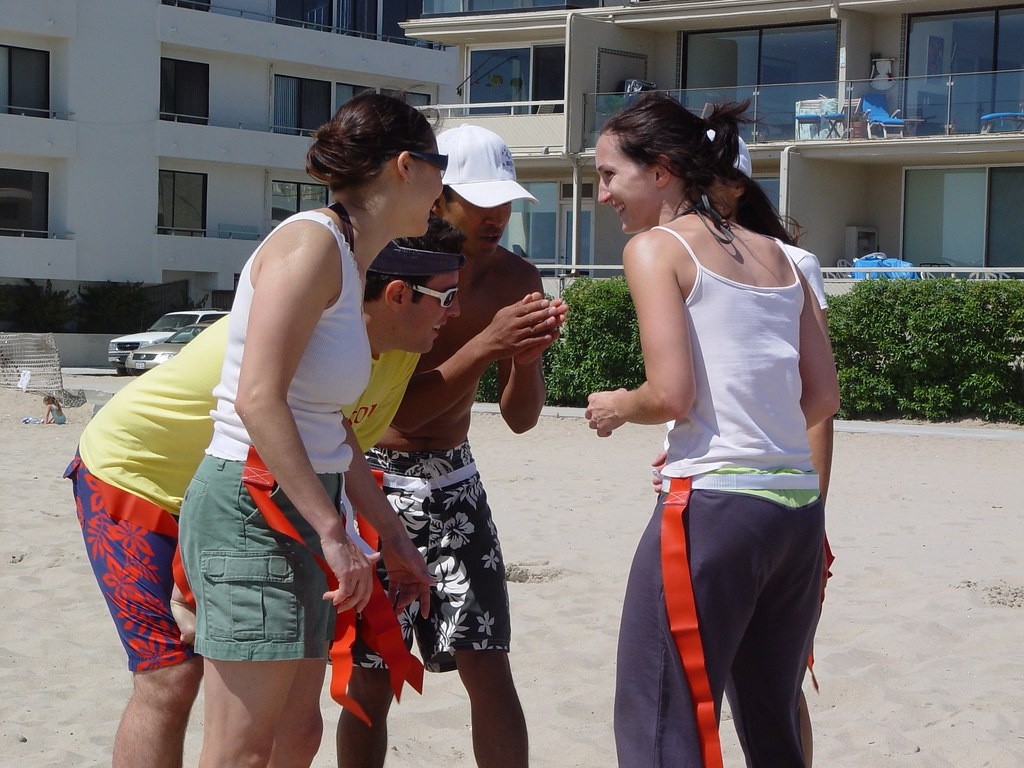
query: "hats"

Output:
[436,124,539,209]
[707,130,752,178]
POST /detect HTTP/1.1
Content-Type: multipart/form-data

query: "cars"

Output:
[125,323,212,375]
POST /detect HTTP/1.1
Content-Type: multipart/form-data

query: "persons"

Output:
[64,207,468,767]
[583,89,842,768]
[177,86,438,768]
[336,125,569,767]
[42,394,66,424]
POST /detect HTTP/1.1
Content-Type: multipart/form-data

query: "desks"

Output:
[902,119,925,137]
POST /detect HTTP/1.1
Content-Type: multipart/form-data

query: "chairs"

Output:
[537,105,555,114]
[796,92,905,140]
[820,253,955,281]
[981,103,1024,134]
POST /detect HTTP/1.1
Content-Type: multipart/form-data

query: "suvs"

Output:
[108,308,231,377]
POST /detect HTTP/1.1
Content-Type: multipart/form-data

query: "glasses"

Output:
[405,281,458,308]
[395,151,448,179]
